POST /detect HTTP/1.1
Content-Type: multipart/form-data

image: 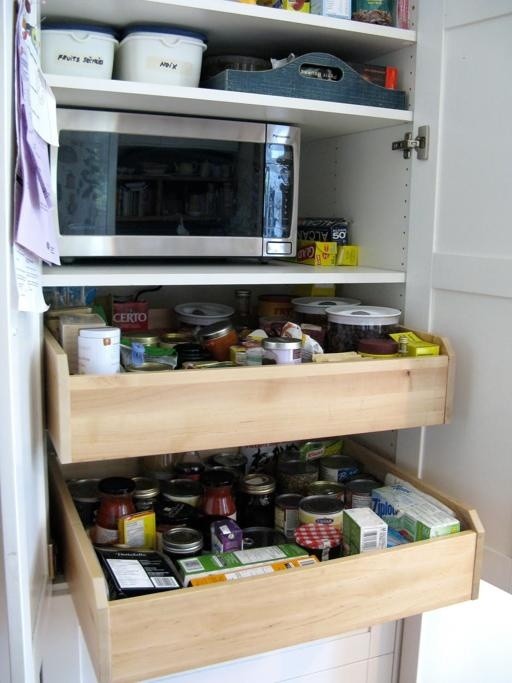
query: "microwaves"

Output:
[49,105,302,262]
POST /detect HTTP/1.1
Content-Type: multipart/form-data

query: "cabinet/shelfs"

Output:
[1,2,484,683]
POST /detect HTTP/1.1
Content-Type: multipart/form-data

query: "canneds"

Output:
[66,451,387,571]
[122,293,302,371]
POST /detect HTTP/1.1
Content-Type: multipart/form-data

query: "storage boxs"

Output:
[38,17,208,89]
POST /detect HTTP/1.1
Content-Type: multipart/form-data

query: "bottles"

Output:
[290,295,401,353]
[78,326,121,376]
[234,289,257,341]
[199,469,275,525]
[94,477,135,545]
